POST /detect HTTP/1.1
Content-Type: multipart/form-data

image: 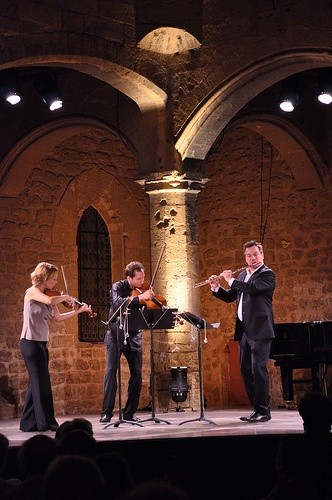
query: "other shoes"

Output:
[47,423,60,432]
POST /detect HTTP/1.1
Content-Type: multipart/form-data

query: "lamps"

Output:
[278,68,332,112]
[4,79,64,112]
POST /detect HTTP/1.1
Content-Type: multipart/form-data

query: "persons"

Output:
[260,391,332,500]
[19,262,91,432]
[208,240,277,422]
[100,261,157,422]
[0,418,189,500]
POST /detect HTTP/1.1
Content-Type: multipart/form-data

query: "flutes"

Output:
[193,261,261,289]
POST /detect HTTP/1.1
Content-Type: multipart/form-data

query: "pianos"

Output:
[268,321,332,409]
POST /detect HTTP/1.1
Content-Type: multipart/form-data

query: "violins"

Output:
[44,286,97,318]
[131,283,184,325]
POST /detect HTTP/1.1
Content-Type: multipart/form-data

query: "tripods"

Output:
[172,311,221,425]
[132,308,178,426]
[102,296,143,428]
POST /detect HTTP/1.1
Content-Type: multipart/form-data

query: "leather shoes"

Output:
[240,411,255,421]
[250,412,271,421]
[99,414,112,422]
[124,414,140,421]
